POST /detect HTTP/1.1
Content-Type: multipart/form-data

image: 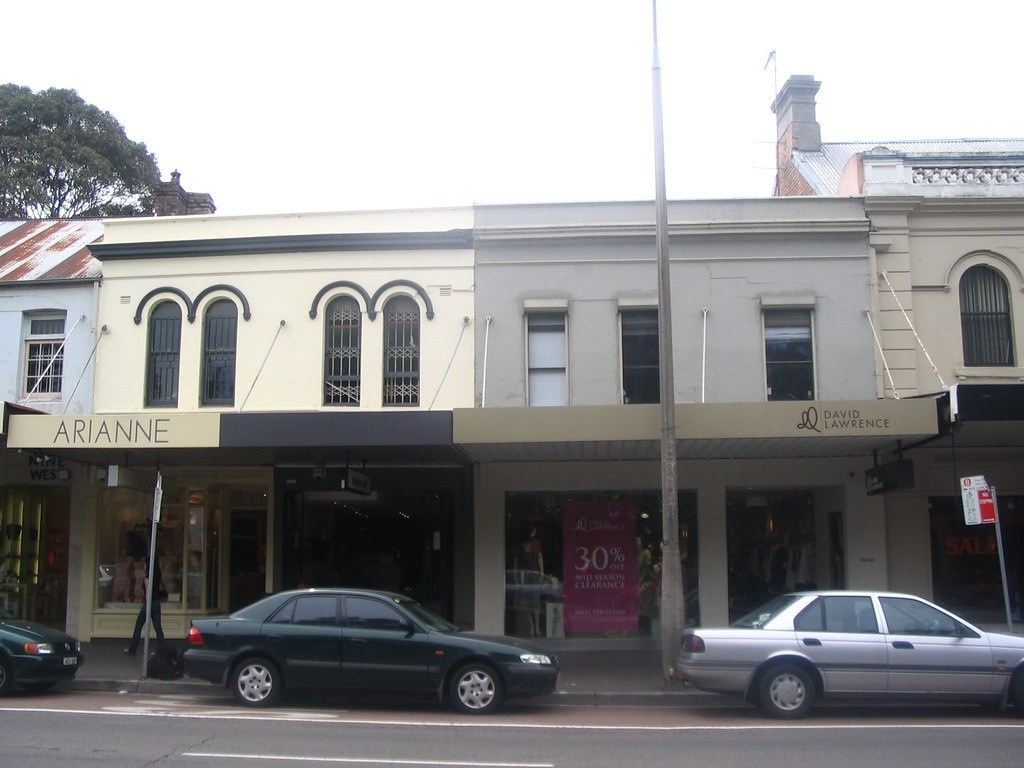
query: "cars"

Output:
[182,587,562,715]
[0,609,87,693]
[505,570,562,608]
[98,565,118,588]
[673,588,1024,720]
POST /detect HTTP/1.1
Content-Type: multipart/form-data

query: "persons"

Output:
[111,545,180,603]
[634,535,657,637]
[122,558,166,656]
[511,524,545,637]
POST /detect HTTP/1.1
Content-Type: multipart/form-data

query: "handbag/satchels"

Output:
[159,589,168,603]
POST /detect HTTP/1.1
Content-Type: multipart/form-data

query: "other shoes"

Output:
[124,648,129,654]
[150,651,156,656]
